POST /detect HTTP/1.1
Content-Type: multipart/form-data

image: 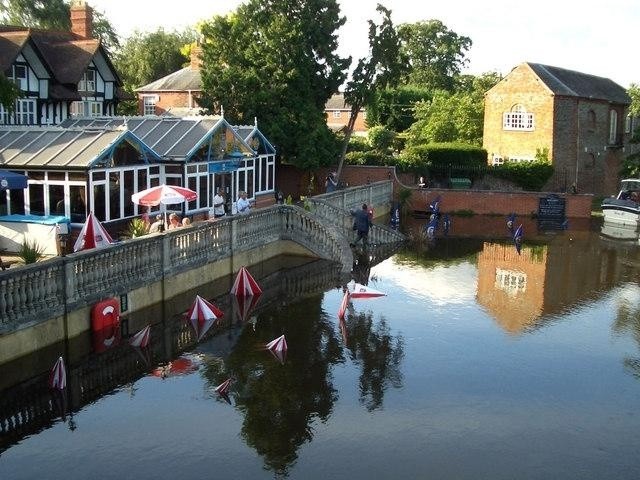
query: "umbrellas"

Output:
[127,324,152,346]
[339,318,350,346]
[188,295,224,320]
[265,334,288,351]
[270,350,287,365]
[351,282,387,298]
[338,290,350,318]
[231,266,262,294]
[49,356,68,389]
[392,195,525,255]
[131,182,198,231]
[51,390,68,424]
[130,347,153,369]
[73,210,113,255]
[231,294,260,323]
[216,378,233,393]
[190,320,215,343]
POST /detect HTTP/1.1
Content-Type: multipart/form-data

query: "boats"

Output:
[600,179,640,226]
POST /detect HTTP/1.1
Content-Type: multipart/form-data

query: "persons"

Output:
[214,187,226,218]
[276,189,311,211]
[133,212,191,237]
[326,170,338,192]
[237,191,253,213]
[349,203,373,249]
